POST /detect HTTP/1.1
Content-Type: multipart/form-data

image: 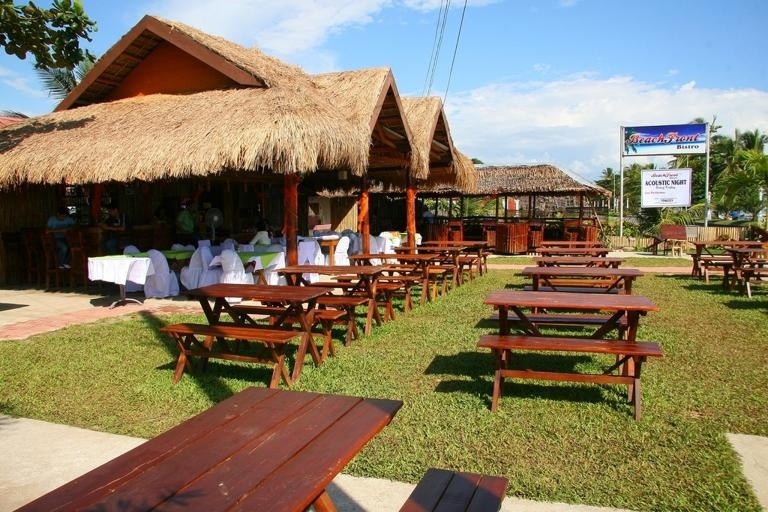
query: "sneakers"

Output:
[56,264,71,268]
